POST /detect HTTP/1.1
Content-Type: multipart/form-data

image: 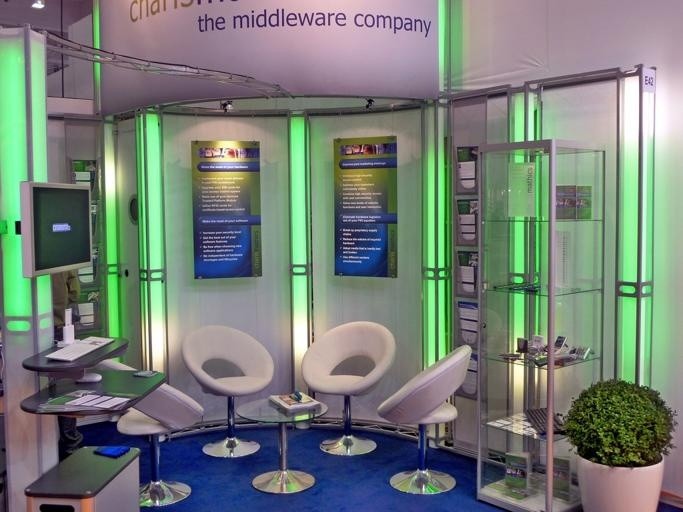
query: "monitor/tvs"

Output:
[19,181,93,278]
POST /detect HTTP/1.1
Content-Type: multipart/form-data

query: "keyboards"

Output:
[44,335,115,363]
[525,407,566,435]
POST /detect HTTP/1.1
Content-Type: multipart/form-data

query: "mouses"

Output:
[77,372,103,383]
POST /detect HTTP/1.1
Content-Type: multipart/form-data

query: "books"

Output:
[552,457,571,500]
[280,392,313,406]
[64,393,130,408]
[487,480,510,492]
[505,450,531,488]
[575,186,591,219]
[556,185,577,219]
[268,389,320,412]
[503,488,537,502]
[46,387,142,412]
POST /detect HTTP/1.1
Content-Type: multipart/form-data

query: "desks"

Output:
[237,394,328,494]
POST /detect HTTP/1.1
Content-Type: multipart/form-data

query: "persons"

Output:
[49,266,82,460]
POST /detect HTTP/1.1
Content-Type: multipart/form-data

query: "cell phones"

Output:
[132,370,156,377]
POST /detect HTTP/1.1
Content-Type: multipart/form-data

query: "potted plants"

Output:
[564,379,679,512]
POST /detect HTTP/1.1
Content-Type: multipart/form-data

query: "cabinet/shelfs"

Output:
[19,333,166,511]
[476,138,606,511]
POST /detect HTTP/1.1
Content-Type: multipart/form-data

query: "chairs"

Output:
[302,321,396,457]
[95,357,206,508]
[181,325,275,457]
[377,345,471,494]
